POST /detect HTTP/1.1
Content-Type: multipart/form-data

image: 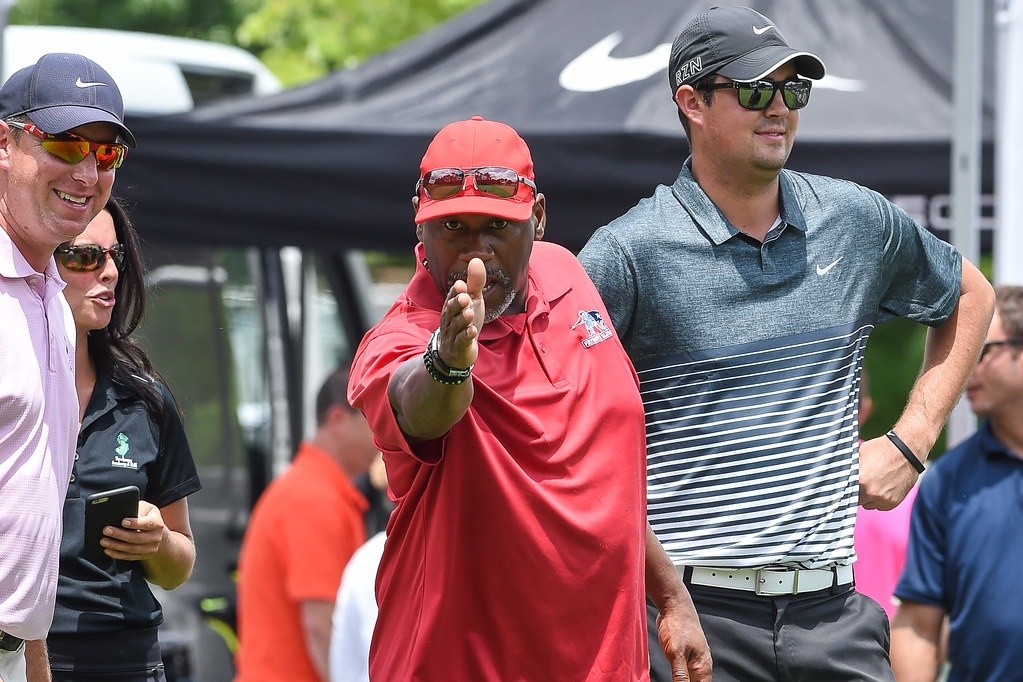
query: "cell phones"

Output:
[82,486,140,573]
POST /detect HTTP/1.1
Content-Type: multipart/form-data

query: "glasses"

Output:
[415,165,537,201]
[53,243,130,274]
[978,336,1023,363]
[692,76,812,111]
[8,120,130,171]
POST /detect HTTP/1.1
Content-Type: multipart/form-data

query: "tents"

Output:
[114,0,1009,269]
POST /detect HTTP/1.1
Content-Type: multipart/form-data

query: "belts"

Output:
[666,564,857,598]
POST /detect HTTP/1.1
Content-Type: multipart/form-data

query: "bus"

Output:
[3,24,383,680]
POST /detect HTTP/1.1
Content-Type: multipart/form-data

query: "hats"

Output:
[668,6,826,97]
[414,116,536,224]
[0,52,140,151]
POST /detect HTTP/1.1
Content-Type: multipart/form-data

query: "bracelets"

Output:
[883,428,926,475]
[422,327,476,386]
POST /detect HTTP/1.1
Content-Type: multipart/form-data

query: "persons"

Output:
[46,195,205,682]
[0,51,137,682]
[346,115,713,682]
[854,284,1023,682]
[234,360,397,682]
[574,4,997,682]
